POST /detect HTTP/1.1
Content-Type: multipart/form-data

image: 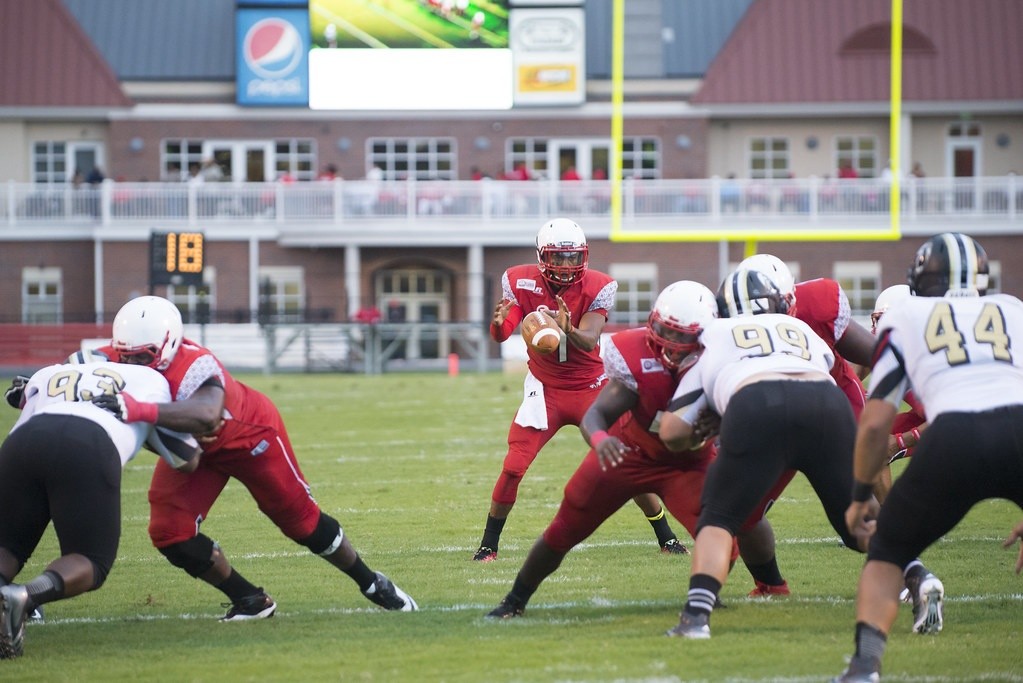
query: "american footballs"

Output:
[521,310,562,353]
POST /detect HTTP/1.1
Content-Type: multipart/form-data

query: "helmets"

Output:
[737,253,802,318]
[111,295,183,374]
[715,267,785,317]
[870,284,917,335]
[535,218,596,287]
[64,348,110,364]
[908,233,990,297]
[648,280,718,368]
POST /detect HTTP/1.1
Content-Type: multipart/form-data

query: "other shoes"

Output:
[0,585,29,659]
[827,655,880,683]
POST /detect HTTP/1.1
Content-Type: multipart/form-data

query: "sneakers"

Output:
[747,581,790,596]
[662,540,690,554]
[663,611,710,638]
[487,598,523,619]
[909,572,945,635]
[218,587,276,623]
[360,570,419,611]
[471,547,497,562]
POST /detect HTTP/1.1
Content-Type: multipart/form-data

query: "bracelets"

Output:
[910,425,921,441]
[894,426,906,454]
[848,477,877,503]
[589,431,608,448]
[689,440,706,451]
[567,324,574,337]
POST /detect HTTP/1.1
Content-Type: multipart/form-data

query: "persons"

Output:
[471,219,690,562]
[0,295,421,659]
[70,152,928,221]
[485,230,1023,683]
